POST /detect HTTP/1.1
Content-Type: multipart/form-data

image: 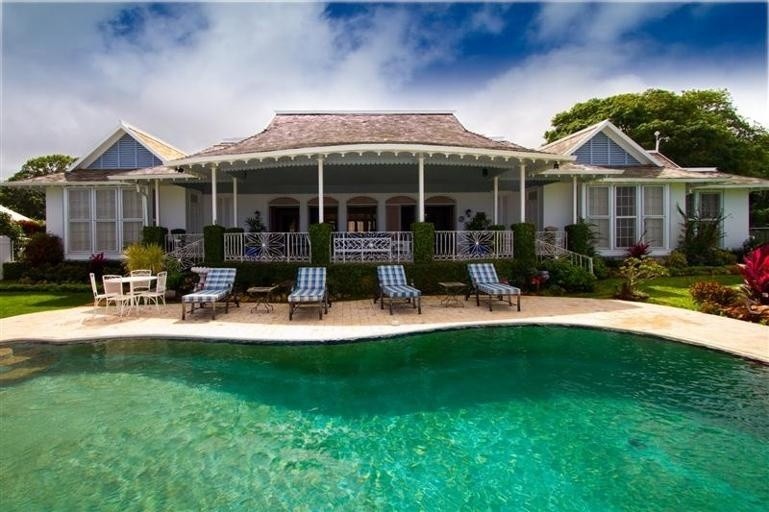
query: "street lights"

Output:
[654,131,670,152]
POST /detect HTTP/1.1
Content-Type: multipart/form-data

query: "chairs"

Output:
[466,263,522,312]
[288,265,331,321]
[376,264,421,314]
[90,269,168,321]
[179,266,239,322]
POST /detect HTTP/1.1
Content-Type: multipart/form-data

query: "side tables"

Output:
[439,281,466,308]
[247,285,276,314]
